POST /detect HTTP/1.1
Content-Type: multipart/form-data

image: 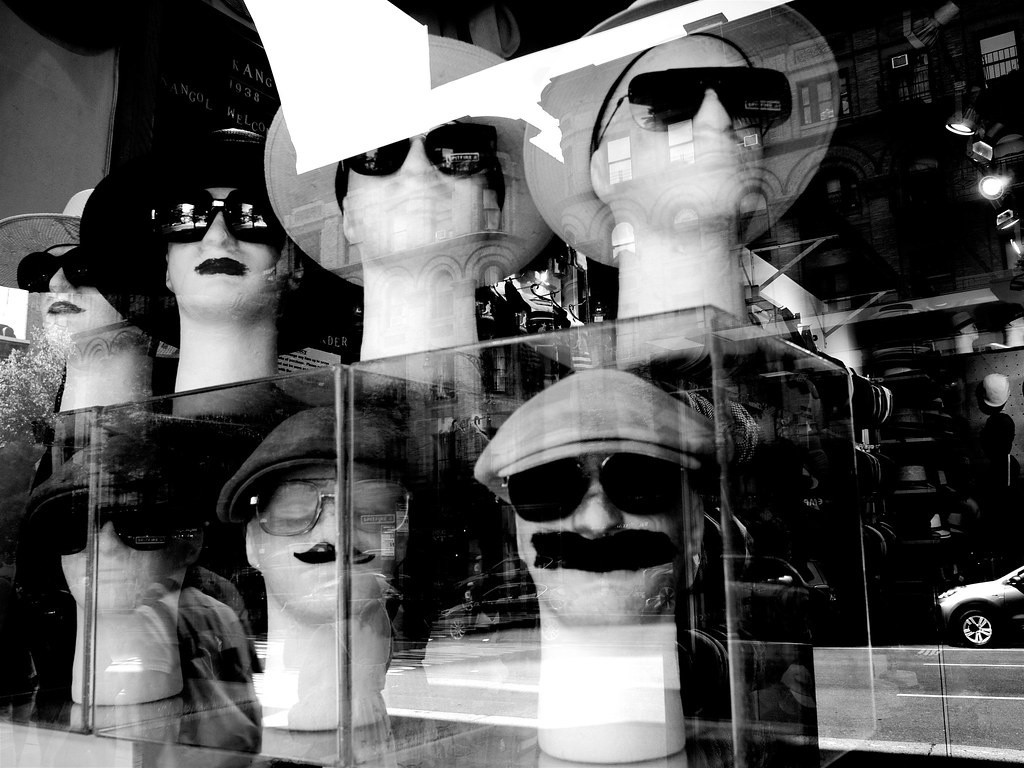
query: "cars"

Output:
[937,563,1024,648]
[436,552,548,639]
[711,552,838,634]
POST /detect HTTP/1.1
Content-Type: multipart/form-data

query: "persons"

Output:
[475,365,716,765]
[24,439,212,703]
[217,404,412,730]
[587,32,773,366]
[340,109,508,393]
[163,159,306,419]
[40,186,156,439]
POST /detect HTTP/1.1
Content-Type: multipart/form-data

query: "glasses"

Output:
[593,66,793,151]
[33,501,177,555]
[250,480,414,537]
[17,246,96,293]
[336,125,505,214]
[149,188,287,250]
[501,453,692,523]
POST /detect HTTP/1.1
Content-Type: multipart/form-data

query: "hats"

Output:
[79,130,358,355]
[0,189,96,290]
[25,435,212,527]
[523,0,841,267]
[473,369,733,504]
[263,34,555,288]
[215,406,418,523]
